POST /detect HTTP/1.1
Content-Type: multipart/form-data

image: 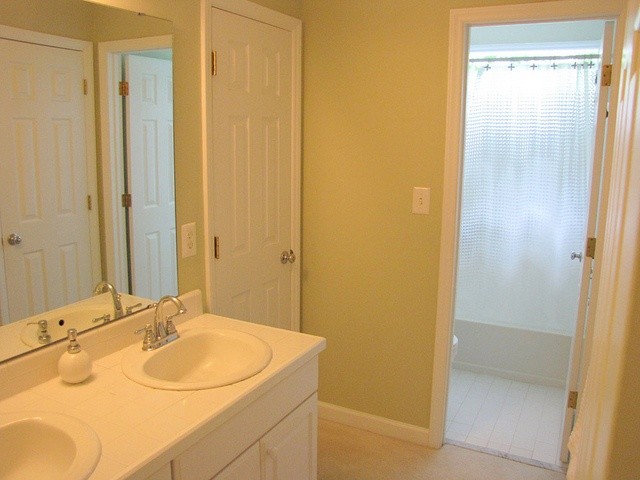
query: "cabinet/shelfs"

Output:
[144,352,319,479]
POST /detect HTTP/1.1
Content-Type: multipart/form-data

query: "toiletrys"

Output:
[26,320,52,345]
[58,327,92,383]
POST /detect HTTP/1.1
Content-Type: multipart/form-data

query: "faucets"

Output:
[93,281,123,319]
[155,294,188,339]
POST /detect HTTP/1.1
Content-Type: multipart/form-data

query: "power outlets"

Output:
[180,221,197,259]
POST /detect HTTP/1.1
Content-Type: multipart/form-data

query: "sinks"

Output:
[21,304,152,352]
[120,327,273,391]
[0,411,103,480]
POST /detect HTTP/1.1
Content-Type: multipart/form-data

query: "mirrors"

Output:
[0,0,179,364]
